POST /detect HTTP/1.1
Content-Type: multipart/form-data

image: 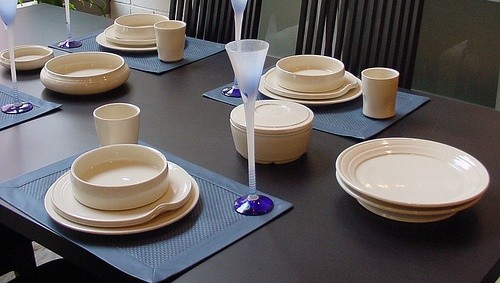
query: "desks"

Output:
[0,3,500,283]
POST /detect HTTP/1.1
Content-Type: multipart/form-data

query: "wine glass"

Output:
[225,39,274,215]
[57,0,82,48]
[0,0,33,114]
[222,0,249,97]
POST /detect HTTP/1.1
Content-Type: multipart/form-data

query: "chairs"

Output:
[168,0,263,50]
[295,0,425,90]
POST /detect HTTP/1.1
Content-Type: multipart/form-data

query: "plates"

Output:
[44,162,200,235]
[258,68,362,104]
[94,24,157,51]
[334,138,490,222]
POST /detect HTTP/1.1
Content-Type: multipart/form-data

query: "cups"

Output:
[93,102,140,147]
[361,67,399,119]
[154,20,186,62]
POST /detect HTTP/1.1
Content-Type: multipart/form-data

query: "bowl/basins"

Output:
[113,13,169,39]
[0,45,54,70]
[229,100,314,164]
[275,54,345,93]
[39,52,130,94]
[70,143,168,211]
